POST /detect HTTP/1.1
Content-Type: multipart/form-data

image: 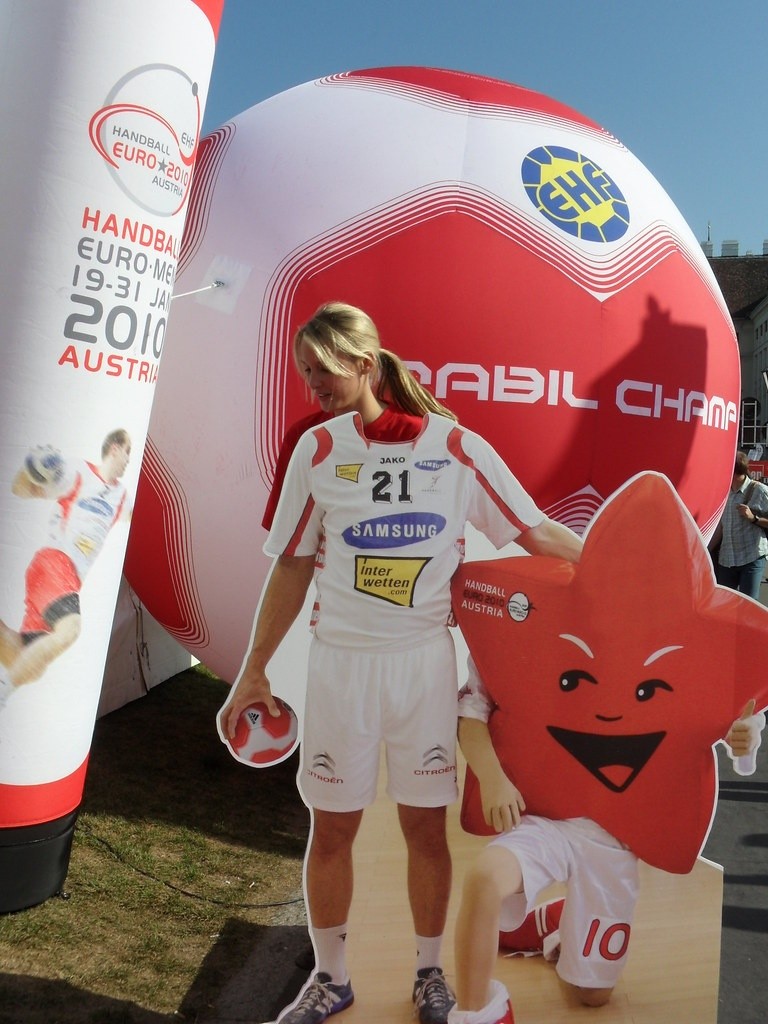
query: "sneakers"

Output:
[279,972,354,1024]
[412,967,457,1024]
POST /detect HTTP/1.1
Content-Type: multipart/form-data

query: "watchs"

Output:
[752,515,759,523]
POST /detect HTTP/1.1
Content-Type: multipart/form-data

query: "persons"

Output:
[0,430,130,708]
[709,452,768,602]
[218,412,765,1024]
[260,302,458,534]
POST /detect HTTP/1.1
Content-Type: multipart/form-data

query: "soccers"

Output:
[226,694,299,764]
[25,446,67,490]
